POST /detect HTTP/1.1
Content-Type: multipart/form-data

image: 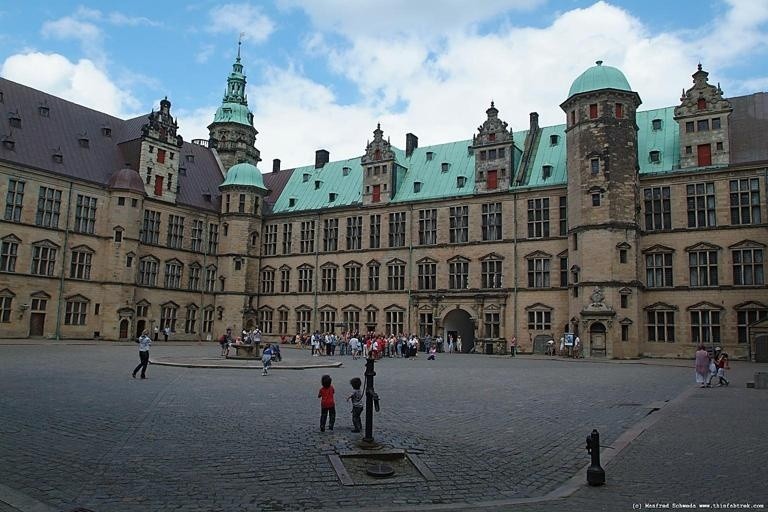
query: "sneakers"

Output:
[320,423,363,432]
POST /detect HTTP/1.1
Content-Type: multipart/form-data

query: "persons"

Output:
[695,344,730,388]
[546,338,555,357]
[346,377,364,433]
[573,334,581,359]
[132,329,152,380]
[558,334,565,357]
[163,324,170,342]
[510,335,517,357]
[221,328,463,376]
[318,375,336,432]
[154,326,159,341]
